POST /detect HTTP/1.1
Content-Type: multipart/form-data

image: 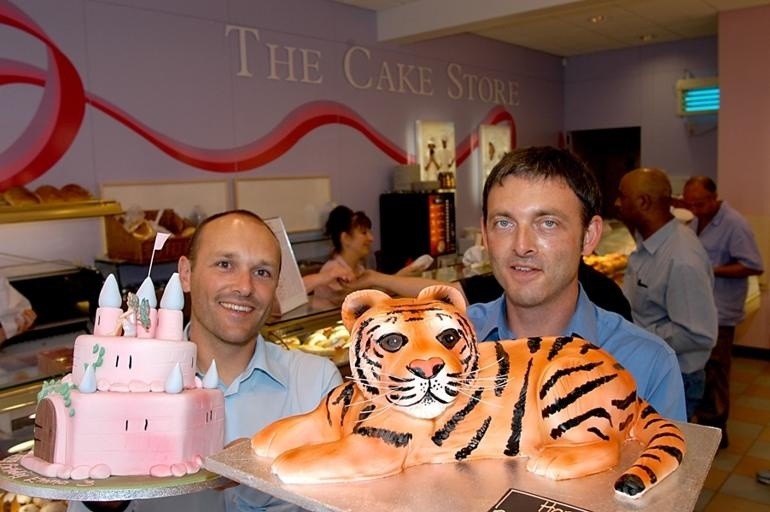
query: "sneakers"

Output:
[757,470,770,485]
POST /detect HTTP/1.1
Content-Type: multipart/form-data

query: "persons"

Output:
[684,175,764,449]
[313,206,426,295]
[338,256,632,320]
[0,309,38,343]
[66,208,343,512]
[465,145,688,421]
[614,167,719,422]
[302,266,357,294]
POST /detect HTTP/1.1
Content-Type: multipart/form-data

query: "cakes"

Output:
[0,491,69,512]
[251,285,688,500]
[20,272,226,482]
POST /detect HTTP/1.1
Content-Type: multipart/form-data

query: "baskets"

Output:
[105,209,195,264]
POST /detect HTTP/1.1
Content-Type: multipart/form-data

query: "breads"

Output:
[581,250,631,285]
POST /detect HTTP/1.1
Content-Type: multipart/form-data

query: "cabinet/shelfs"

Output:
[2,199,125,446]
